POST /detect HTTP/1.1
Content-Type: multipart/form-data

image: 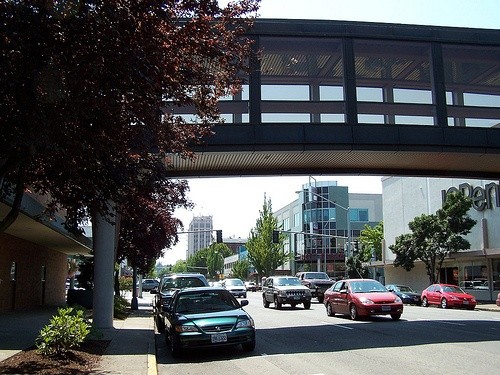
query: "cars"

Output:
[323,277,404,321]
[161,286,257,358]
[136,278,160,292]
[385,284,421,306]
[243,281,257,292]
[420,283,478,311]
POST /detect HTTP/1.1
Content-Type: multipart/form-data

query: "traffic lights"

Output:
[216,229,224,244]
[273,229,280,244]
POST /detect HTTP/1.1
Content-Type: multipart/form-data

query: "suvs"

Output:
[149,276,207,333]
[221,276,247,299]
[459,280,489,290]
[262,275,313,310]
[294,271,337,304]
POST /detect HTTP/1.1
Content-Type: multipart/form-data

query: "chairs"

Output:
[211,295,221,300]
[180,298,190,306]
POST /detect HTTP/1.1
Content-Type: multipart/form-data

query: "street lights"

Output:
[294,190,351,260]
[323,216,336,273]
